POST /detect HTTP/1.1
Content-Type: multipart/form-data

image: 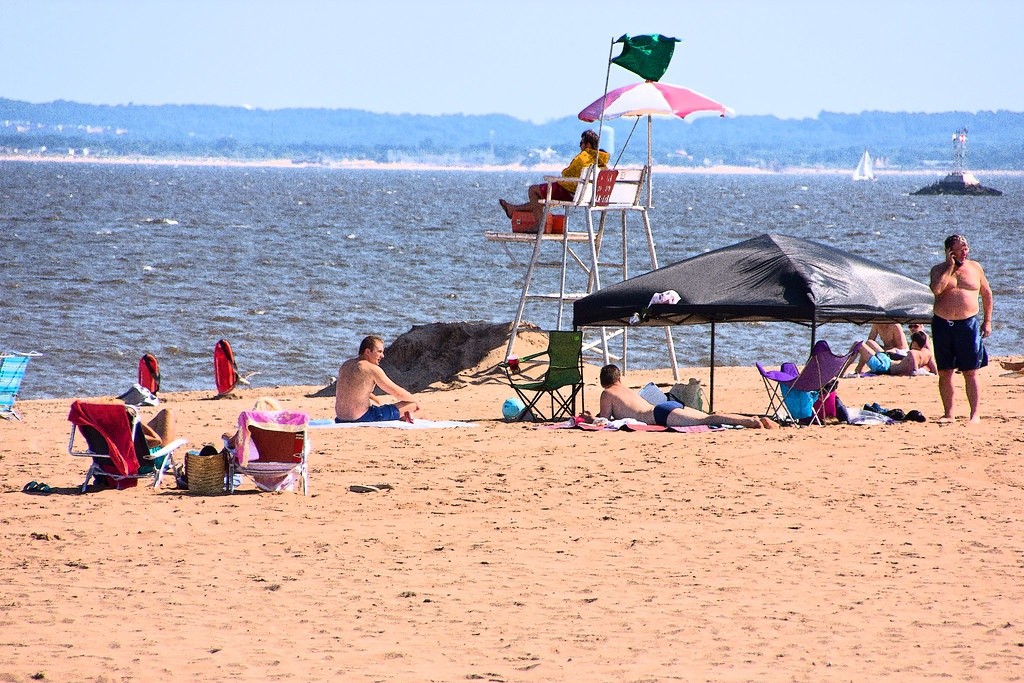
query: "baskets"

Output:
[183,451,225,495]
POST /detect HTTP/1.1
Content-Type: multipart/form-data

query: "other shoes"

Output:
[863,402,926,423]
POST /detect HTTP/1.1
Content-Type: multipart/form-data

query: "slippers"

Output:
[29,483,57,495]
[499,199,512,219]
[18,481,40,493]
[526,229,537,234]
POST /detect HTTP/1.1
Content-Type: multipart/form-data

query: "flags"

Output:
[610,33,683,82]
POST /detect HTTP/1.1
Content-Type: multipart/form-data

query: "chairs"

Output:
[498,331,585,423]
[755,340,862,428]
[221,411,309,496]
[0,349,44,423]
[68,400,187,492]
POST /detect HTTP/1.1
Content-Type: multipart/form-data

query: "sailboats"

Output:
[852,150,877,182]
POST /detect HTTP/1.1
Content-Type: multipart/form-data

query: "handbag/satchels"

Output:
[637,379,709,411]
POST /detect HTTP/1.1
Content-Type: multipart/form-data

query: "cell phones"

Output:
[949,250,963,267]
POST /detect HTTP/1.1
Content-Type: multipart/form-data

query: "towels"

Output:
[237,410,310,467]
[627,288,681,325]
[67,398,140,475]
[667,383,701,411]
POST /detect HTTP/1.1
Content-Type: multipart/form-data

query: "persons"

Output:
[908,325,933,353]
[834,332,938,375]
[335,335,420,423]
[499,129,610,233]
[219,397,283,453]
[867,323,909,350]
[127,408,162,448]
[929,234,993,424]
[595,364,777,429]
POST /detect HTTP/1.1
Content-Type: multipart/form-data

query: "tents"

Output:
[571,233,935,417]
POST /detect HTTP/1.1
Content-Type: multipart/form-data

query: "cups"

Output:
[507,354,519,370]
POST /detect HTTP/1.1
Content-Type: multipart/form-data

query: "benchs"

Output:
[538,164,654,210]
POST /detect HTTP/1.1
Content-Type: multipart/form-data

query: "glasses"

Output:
[908,324,920,328]
[580,141,586,147]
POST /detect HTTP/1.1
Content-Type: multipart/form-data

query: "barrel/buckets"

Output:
[639,382,667,405]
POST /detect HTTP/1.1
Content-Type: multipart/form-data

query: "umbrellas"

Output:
[578,80,727,209]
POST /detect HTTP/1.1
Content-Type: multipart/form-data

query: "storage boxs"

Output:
[512,211,565,234]
